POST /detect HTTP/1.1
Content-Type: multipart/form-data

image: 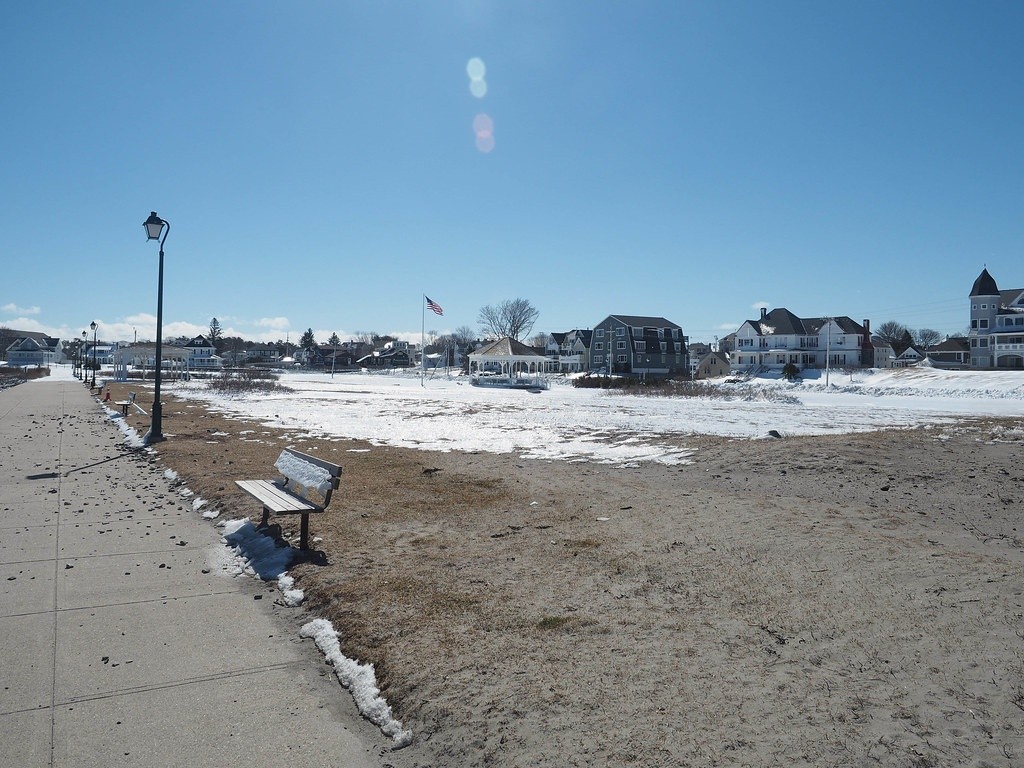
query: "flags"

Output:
[425,295,443,316]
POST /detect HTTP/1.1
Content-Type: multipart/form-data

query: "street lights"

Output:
[142,211,170,443]
[77,339,84,380]
[90,321,98,386]
[75,342,80,378]
[82,331,88,382]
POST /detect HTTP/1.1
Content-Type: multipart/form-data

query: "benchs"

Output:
[114,391,136,417]
[93,381,106,395]
[235,446,343,562]
